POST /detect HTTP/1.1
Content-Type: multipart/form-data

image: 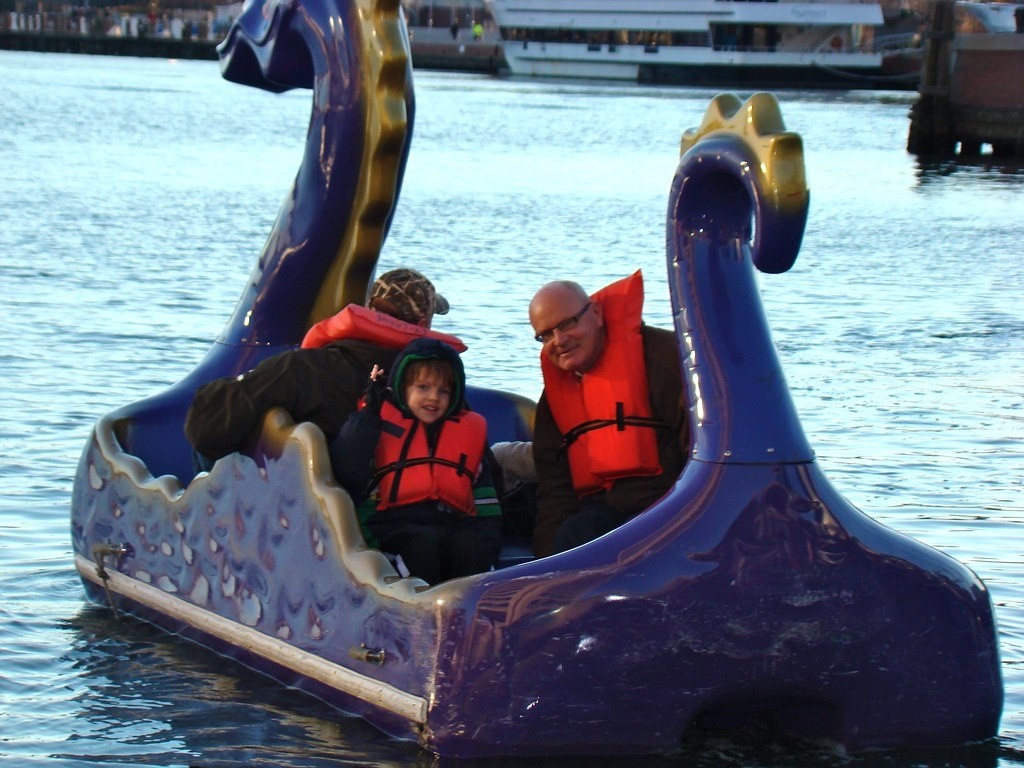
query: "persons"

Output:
[473,21,483,41]
[0,0,233,41]
[329,337,504,588]
[185,268,449,463]
[450,19,459,41]
[529,281,691,561]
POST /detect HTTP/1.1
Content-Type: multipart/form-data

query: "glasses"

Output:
[535,301,592,342]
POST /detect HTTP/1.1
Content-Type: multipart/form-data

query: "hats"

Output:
[368,268,450,325]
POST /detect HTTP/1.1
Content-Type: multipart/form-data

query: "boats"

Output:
[905,1,1024,172]
[485,1,923,87]
[66,1,1008,758]
